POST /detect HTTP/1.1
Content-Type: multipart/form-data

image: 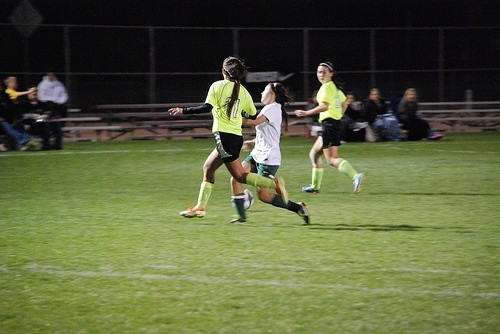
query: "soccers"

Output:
[231,189,253,211]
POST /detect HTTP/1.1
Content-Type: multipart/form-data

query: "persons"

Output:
[312,88,443,142]
[294,62,365,195]
[168,57,310,225]
[0,71,69,151]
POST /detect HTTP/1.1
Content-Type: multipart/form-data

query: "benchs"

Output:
[35,101,500,144]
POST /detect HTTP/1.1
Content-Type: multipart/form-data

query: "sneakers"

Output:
[0,144,7,152]
[352,172,365,194]
[274,177,289,207]
[428,134,443,140]
[301,185,321,194]
[296,202,311,226]
[21,138,42,150]
[229,217,248,226]
[179,207,206,218]
